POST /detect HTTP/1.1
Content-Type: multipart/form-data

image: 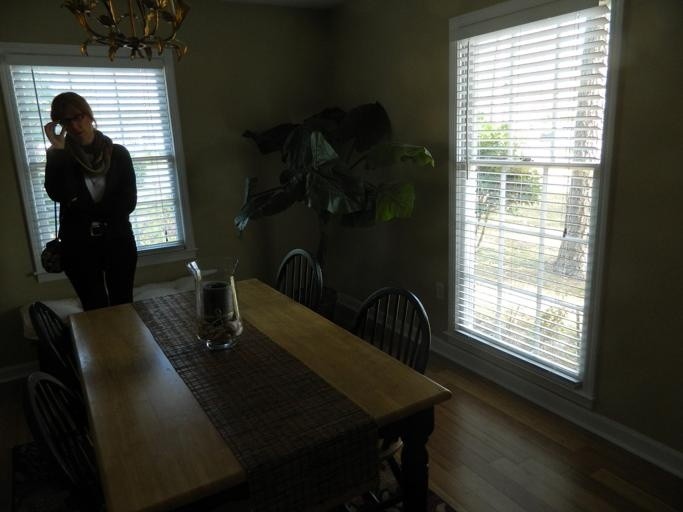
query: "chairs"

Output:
[20,370,105,486]
[349,286,437,500]
[268,249,328,316]
[25,299,74,369]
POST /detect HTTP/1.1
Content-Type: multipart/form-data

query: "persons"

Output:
[43,93,138,311]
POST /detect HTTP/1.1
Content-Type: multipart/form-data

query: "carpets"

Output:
[12,442,459,511]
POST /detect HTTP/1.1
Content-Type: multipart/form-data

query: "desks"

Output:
[66,277,456,511]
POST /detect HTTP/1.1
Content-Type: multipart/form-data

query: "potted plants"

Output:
[235,100,436,321]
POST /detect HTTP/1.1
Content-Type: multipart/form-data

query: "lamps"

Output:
[57,0,194,63]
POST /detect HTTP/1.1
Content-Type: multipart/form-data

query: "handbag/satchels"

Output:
[39,237,73,274]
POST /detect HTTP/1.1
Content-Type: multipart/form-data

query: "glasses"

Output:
[59,113,86,126]
